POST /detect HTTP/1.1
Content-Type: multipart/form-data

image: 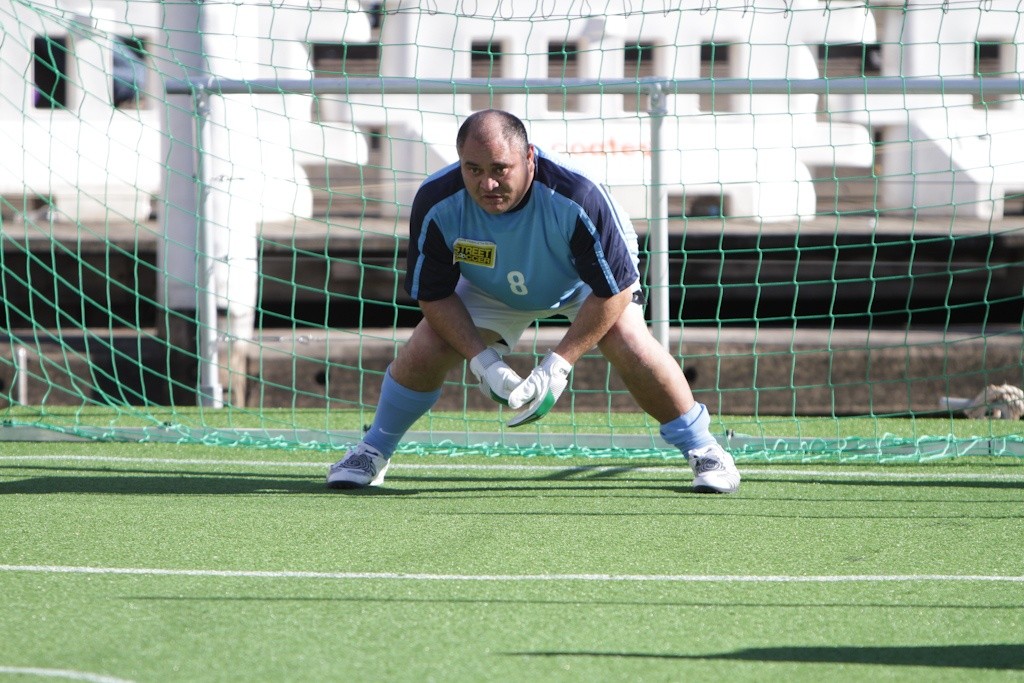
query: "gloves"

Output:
[469,347,531,405]
[506,347,572,428]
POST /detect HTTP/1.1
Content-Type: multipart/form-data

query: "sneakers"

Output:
[688,443,741,492]
[327,442,389,488]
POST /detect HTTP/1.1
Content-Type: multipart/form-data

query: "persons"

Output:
[326,110,741,493]
